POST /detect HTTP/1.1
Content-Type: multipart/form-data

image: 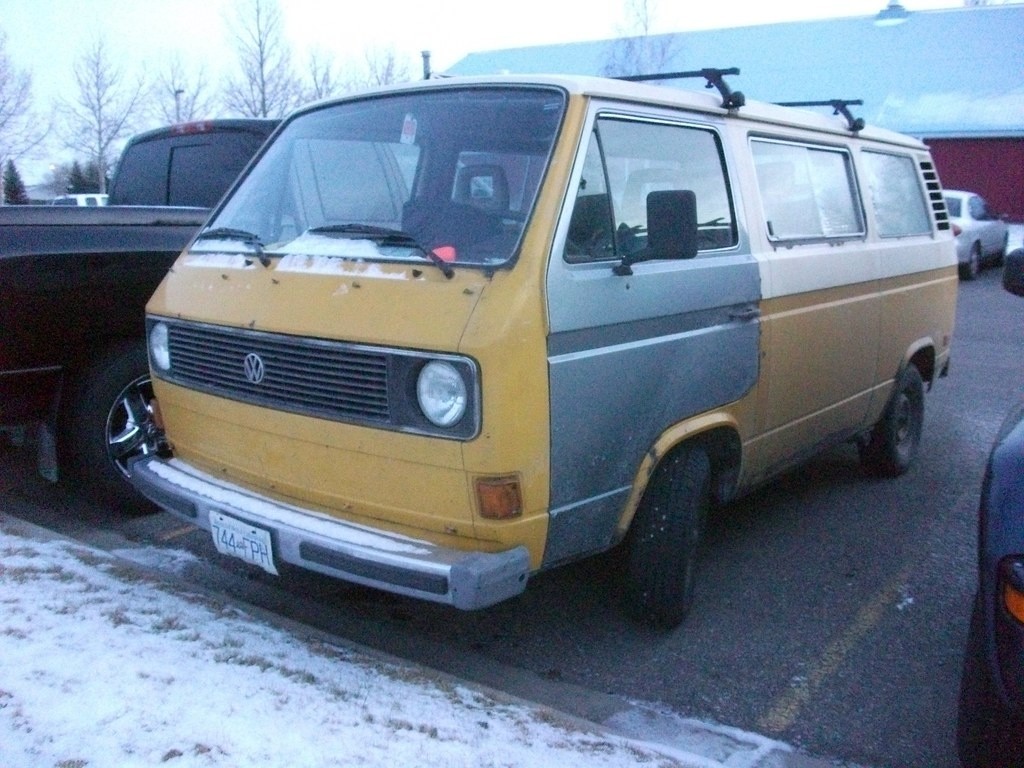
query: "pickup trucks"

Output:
[125,65,960,629]
[1,116,518,518]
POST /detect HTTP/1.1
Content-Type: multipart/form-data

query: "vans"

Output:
[53,194,108,207]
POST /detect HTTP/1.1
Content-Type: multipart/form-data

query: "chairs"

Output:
[592,167,719,258]
[454,163,509,223]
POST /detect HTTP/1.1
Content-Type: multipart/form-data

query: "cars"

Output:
[954,247,1023,768]
[937,189,1009,278]
